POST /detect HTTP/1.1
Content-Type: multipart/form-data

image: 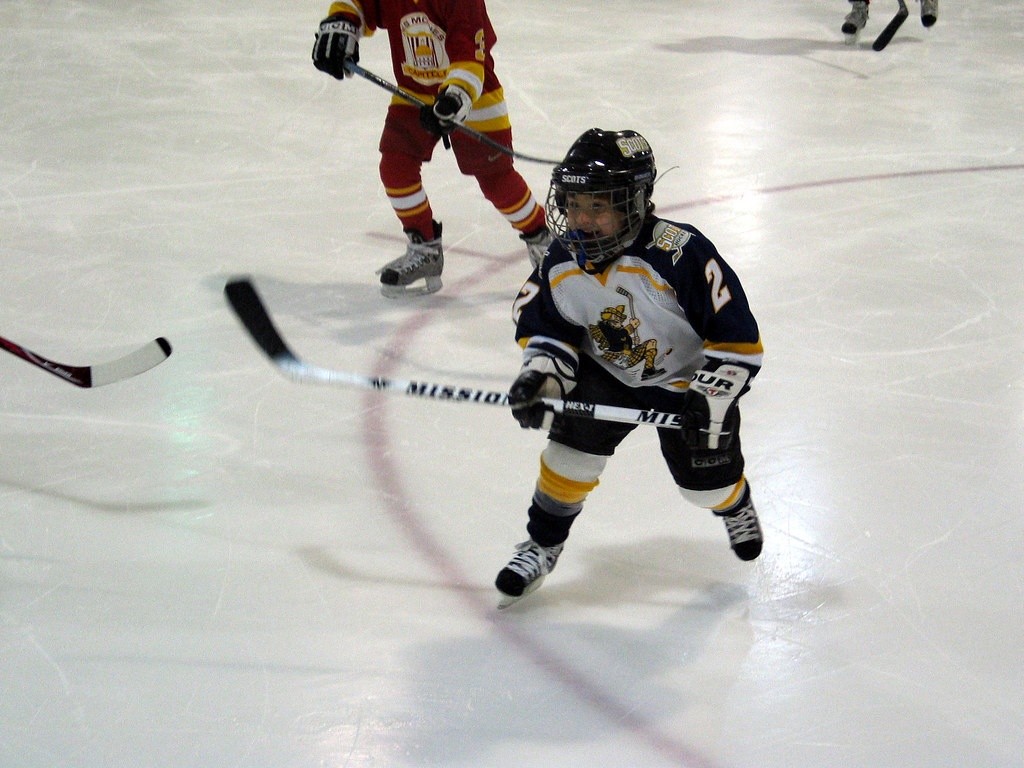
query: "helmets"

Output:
[553,127,656,225]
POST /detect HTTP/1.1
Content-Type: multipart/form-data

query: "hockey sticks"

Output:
[0,334,173,389]
[344,60,562,165]
[871,0,909,51]
[223,275,681,429]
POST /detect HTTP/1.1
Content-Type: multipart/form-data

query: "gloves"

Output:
[509,369,556,430]
[431,85,472,135]
[312,10,362,82]
[683,389,737,459]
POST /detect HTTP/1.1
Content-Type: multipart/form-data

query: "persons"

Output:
[496,128,765,610]
[313,0,558,296]
[836,0,942,34]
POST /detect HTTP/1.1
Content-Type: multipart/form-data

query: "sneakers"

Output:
[520,225,554,271]
[376,218,443,297]
[723,498,763,560]
[916,0,938,27]
[495,537,564,609]
[841,0,869,45]
